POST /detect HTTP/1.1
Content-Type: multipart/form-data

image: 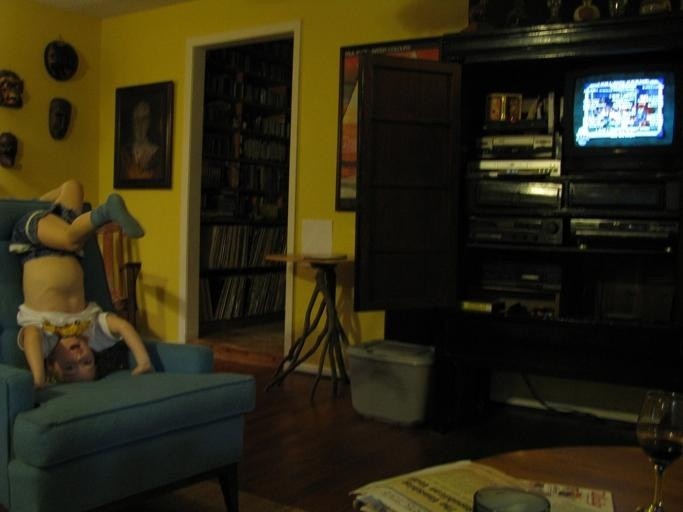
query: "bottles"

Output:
[468,0,672,32]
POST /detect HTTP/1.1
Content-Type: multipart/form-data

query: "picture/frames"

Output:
[113,80,174,189]
[335,35,441,211]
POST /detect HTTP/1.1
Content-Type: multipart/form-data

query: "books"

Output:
[200,44,293,321]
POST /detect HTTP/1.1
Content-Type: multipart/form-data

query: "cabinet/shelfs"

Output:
[198,40,294,337]
[353,9,683,434]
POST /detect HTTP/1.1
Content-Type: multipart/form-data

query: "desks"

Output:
[262,254,358,401]
[363,444,683,512]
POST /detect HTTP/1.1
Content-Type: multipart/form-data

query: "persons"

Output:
[7,178,155,391]
[117,99,162,179]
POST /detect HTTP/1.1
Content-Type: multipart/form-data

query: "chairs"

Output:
[95,222,141,328]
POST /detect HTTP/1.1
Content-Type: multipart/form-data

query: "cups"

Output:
[472,486,551,511]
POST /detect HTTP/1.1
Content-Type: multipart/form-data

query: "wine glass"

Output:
[636,391,682,512]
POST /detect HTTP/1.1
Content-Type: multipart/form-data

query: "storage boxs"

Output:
[346,338,436,426]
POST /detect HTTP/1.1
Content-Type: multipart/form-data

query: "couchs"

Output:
[0,198,256,512]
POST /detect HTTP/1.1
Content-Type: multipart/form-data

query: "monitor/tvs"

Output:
[564,67,682,178]
